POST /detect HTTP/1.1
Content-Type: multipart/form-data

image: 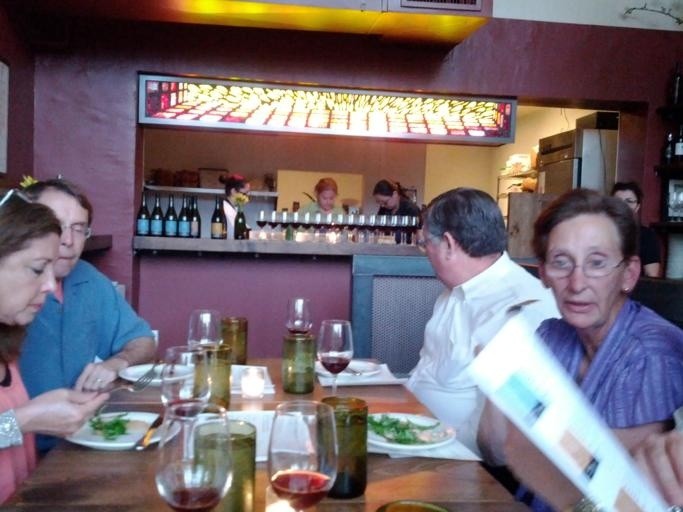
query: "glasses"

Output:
[376,197,389,206]
[0,188,33,207]
[541,252,627,280]
[415,234,441,256]
[59,224,92,240]
[624,199,637,206]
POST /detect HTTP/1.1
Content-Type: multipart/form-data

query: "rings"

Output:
[94,377,103,384]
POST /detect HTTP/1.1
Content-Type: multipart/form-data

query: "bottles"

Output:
[234,203,245,240]
[190,195,201,237]
[210,195,223,238]
[150,192,163,236]
[136,192,150,236]
[164,193,178,237]
[178,193,190,237]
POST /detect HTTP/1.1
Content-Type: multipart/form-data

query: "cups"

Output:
[290,212,300,241]
[268,400,339,507]
[667,191,677,217]
[267,211,279,240]
[194,346,233,412]
[677,192,683,216]
[300,212,313,241]
[319,397,368,498]
[283,334,315,394]
[357,214,368,243]
[313,213,323,241]
[193,422,257,507]
[344,214,356,242]
[333,213,344,242]
[223,317,248,364]
[400,215,411,245]
[318,319,354,396]
[377,215,388,244]
[287,297,313,333]
[155,404,235,508]
[256,210,267,239]
[368,214,377,243]
[161,346,211,460]
[323,213,333,242]
[411,216,419,245]
[279,211,290,240]
[188,310,223,349]
[388,215,400,244]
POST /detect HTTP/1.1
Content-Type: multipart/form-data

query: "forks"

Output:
[110,372,152,394]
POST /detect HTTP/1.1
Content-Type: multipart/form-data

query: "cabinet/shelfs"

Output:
[134,235,619,358]
[635,106,683,325]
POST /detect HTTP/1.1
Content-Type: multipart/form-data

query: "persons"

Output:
[610,179,658,279]
[401,188,541,496]
[634,431,683,512]
[218,174,250,241]
[15,179,157,461]
[476,187,682,512]
[372,178,409,218]
[296,177,346,214]
[0,172,110,508]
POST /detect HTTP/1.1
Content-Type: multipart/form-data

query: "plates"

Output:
[184,411,315,465]
[67,411,181,451]
[316,361,381,378]
[366,413,456,453]
[118,362,194,386]
